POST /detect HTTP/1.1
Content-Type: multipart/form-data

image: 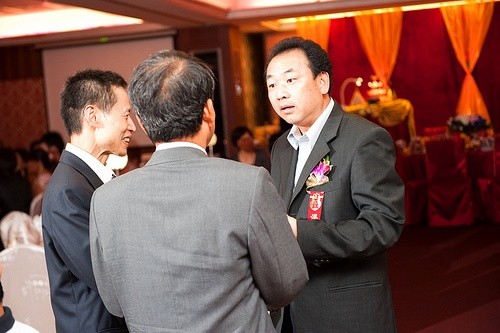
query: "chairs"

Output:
[394,126,500,226]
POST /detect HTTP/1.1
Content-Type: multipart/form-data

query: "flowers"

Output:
[304,155,333,192]
[448,113,487,133]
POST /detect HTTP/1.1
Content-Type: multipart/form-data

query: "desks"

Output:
[412,149,493,180]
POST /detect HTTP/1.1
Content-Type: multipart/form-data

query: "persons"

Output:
[263,37,407,333]
[224,126,272,170]
[0,130,63,333]
[39,69,136,333]
[90,49,311,333]
